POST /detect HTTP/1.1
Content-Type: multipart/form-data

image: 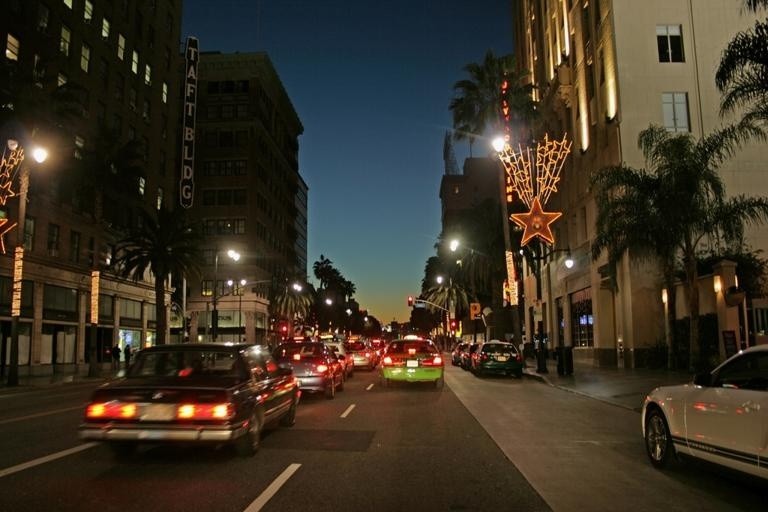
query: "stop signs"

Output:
[468,301,484,322]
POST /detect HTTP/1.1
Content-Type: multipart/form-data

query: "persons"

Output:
[112,343,122,370]
[124,344,131,369]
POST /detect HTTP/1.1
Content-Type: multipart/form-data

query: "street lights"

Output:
[225,279,247,342]
[435,275,489,344]
[484,136,555,372]
[532,244,575,374]
[209,245,242,343]
[7,139,48,389]
[450,238,497,332]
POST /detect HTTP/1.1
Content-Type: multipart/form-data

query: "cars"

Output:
[327,338,386,373]
[639,344,768,483]
[472,338,526,377]
[381,334,445,388]
[273,341,345,400]
[451,341,477,368]
[75,342,302,463]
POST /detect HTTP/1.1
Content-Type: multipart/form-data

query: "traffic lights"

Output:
[276,320,289,339]
[406,294,415,306]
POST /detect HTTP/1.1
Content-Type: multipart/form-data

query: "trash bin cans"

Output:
[555,346,575,376]
